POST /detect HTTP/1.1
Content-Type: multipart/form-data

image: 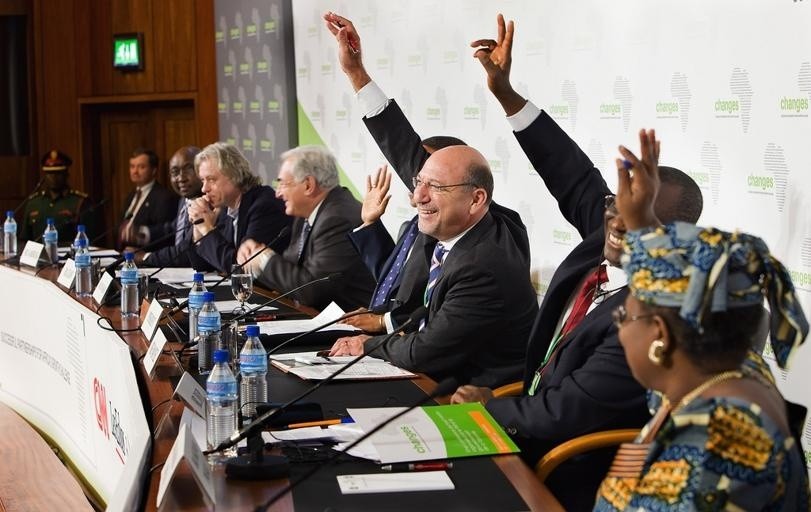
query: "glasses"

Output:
[605,193,616,208]
[612,306,659,327]
[412,176,479,193]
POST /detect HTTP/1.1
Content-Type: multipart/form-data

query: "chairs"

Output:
[491,380,639,483]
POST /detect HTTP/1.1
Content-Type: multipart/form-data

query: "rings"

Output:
[342,340,353,346]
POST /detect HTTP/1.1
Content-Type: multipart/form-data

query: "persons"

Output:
[119,147,204,268]
[188,144,293,276]
[17,149,99,249]
[318,9,540,393]
[337,136,530,337]
[448,11,704,511]
[592,123,805,512]
[237,146,377,313]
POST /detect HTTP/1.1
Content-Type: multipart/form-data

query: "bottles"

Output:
[76,239,92,296]
[189,274,208,344]
[198,294,222,374]
[43,219,58,267]
[74,226,89,252]
[3,211,18,254]
[206,350,240,466]
[238,325,269,417]
[121,254,140,320]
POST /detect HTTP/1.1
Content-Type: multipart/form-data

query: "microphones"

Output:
[220,302,428,479]
[118,219,229,313]
[158,223,293,347]
[57,212,134,272]
[177,268,348,389]
[227,301,399,382]
[249,375,463,512]
[97,216,206,278]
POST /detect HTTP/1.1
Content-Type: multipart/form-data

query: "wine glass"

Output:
[231,265,253,316]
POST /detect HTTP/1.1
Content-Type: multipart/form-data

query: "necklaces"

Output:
[653,371,749,414]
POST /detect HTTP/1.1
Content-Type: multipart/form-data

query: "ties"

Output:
[372,218,418,308]
[541,266,609,377]
[419,243,446,331]
[174,205,186,245]
[130,190,142,213]
[298,221,309,264]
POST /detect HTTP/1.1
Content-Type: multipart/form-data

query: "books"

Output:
[331,399,524,467]
[268,348,420,380]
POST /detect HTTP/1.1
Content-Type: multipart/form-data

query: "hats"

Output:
[42,151,73,174]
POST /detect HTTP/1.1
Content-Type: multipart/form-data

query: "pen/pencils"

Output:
[288,418,355,428]
[239,314,281,322]
[381,462,453,472]
